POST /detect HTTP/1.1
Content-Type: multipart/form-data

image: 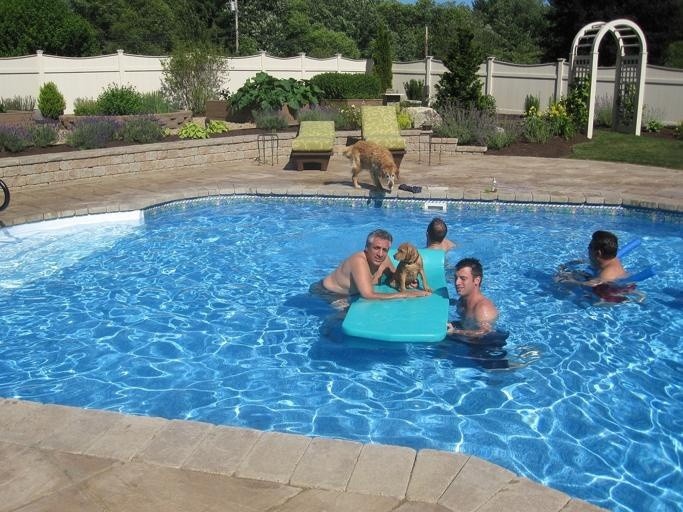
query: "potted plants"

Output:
[308,72,382,107]
[291,106,406,170]
[256,116,286,134]
[53,82,193,131]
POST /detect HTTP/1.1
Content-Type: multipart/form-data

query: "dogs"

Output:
[385,243,432,295]
[343,140,399,193]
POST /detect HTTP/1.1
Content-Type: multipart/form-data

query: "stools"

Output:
[257,135,279,167]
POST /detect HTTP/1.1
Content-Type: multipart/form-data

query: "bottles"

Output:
[491,175,497,192]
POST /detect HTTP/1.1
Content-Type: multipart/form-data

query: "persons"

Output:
[442,257,499,338]
[308,228,431,304]
[558,230,627,289]
[424,217,457,252]
